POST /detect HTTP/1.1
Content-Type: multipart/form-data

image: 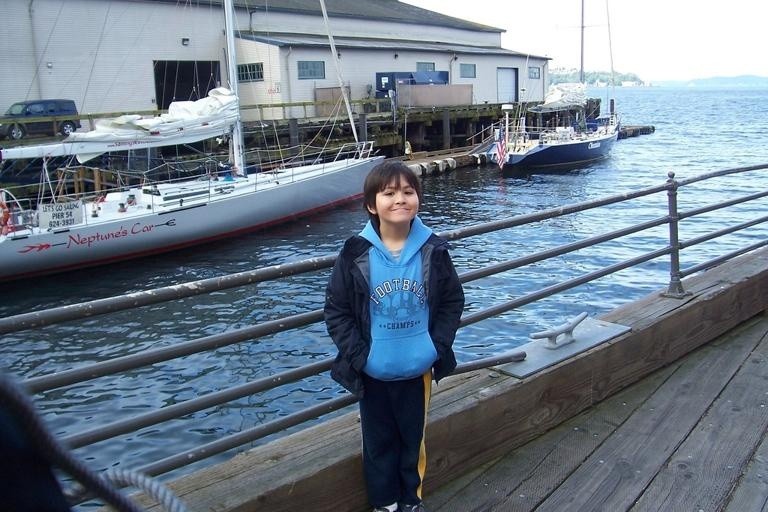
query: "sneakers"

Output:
[372,503,425,512]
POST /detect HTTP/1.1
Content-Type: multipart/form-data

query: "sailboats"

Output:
[490,0,619,177]
[0,0,385,284]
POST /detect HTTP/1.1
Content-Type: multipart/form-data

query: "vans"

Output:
[0,97,81,139]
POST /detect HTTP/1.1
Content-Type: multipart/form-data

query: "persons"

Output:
[323,160,466,512]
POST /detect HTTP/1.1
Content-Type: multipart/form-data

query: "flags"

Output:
[496,127,507,172]
